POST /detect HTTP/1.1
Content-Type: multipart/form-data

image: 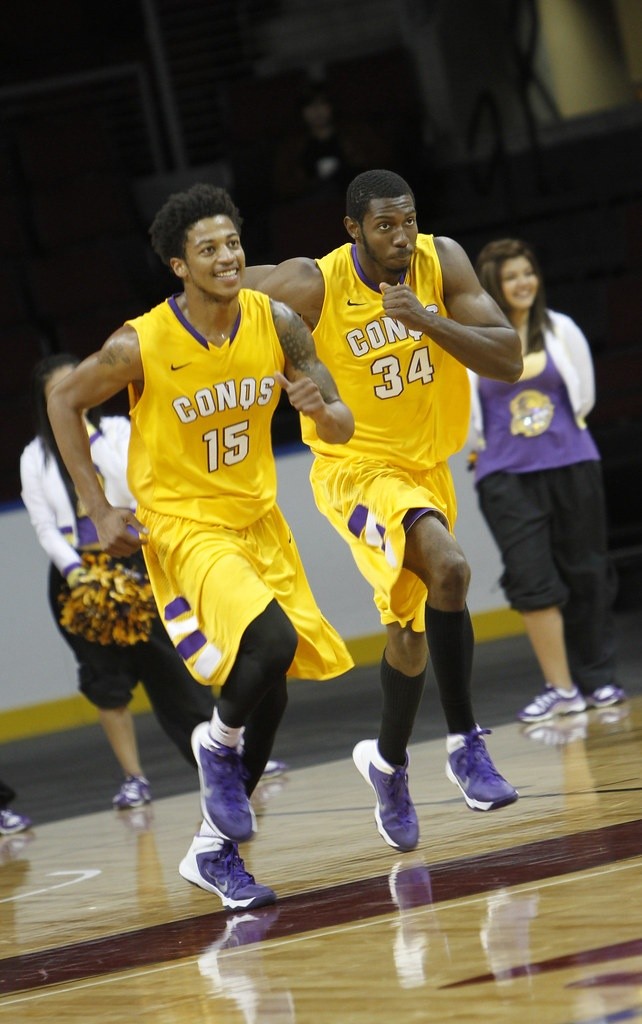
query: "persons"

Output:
[20,348,285,811]
[244,170,525,851]
[465,239,626,722]
[47,185,356,911]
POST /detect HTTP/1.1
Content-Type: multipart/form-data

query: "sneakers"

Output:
[249,776,289,818]
[583,684,624,710]
[444,721,520,813]
[112,776,152,807]
[0,831,35,867]
[351,737,420,852]
[190,720,259,844]
[261,760,285,781]
[524,712,590,749]
[0,807,33,835]
[480,885,540,988]
[197,905,283,981]
[178,833,276,909]
[389,853,453,989]
[117,801,156,837]
[518,684,588,723]
[598,700,632,726]
